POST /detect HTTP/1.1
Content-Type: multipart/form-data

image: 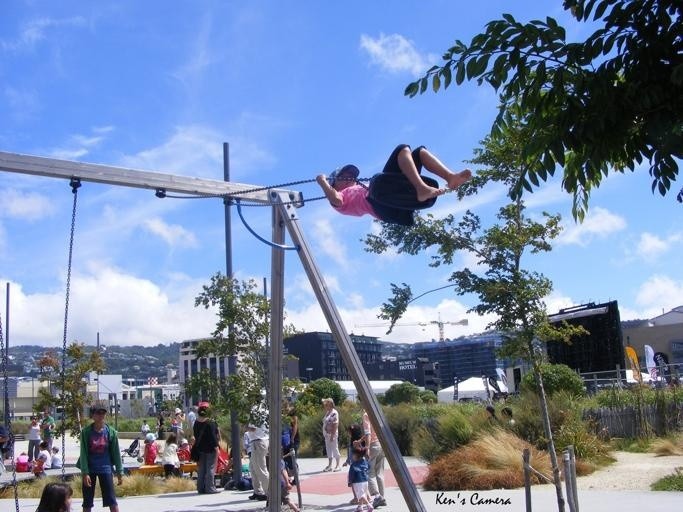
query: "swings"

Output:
[163,172,439,211]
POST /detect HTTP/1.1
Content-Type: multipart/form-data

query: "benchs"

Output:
[128,463,198,481]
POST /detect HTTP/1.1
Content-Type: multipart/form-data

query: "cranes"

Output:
[360,313,468,339]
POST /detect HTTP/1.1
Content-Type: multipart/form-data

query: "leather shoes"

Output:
[214,488,224,493]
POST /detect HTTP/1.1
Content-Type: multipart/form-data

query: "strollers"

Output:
[121,436,143,464]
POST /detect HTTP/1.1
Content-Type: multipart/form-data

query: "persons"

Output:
[358,395,386,509]
[35,482,73,512]
[76,401,126,512]
[485,405,500,425]
[349,441,374,512]
[342,424,370,504]
[317,144,472,227]
[17,409,61,471]
[501,408,516,426]
[321,397,341,472]
[140,398,302,512]
[0,422,10,489]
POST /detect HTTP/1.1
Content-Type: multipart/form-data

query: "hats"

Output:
[145,433,156,442]
[322,398,335,408]
[174,408,181,415]
[180,439,188,446]
[88,404,108,418]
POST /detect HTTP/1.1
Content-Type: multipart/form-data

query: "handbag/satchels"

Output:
[243,476,253,490]
[0,436,13,453]
[190,439,201,462]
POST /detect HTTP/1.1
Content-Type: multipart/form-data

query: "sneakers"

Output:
[323,466,333,472]
[350,493,386,512]
[333,467,341,472]
[248,493,257,500]
[257,495,267,501]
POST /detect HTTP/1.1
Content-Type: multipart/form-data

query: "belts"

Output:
[250,439,260,442]
